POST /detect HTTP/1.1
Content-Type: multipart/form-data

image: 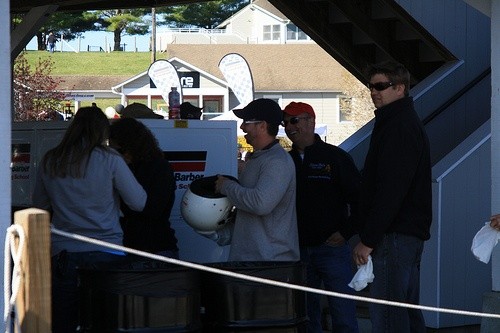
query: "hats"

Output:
[282,102,316,117]
[122,103,164,119]
[233,98,282,124]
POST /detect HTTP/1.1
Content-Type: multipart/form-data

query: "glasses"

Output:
[281,117,309,127]
[368,82,398,91]
[243,120,262,125]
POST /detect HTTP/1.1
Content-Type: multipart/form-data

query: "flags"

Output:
[147,59,183,106]
[218,53,254,107]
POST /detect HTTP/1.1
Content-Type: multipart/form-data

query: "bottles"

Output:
[169,87,180,119]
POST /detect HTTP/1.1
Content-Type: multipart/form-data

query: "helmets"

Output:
[181,175,241,234]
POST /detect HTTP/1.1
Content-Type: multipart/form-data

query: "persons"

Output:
[109,117,180,269]
[36,107,147,333]
[353,61,433,333]
[196,98,300,261]
[490,214,500,232]
[280,102,363,333]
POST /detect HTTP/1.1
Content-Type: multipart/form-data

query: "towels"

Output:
[347,255,375,291]
[471,221,500,264]
